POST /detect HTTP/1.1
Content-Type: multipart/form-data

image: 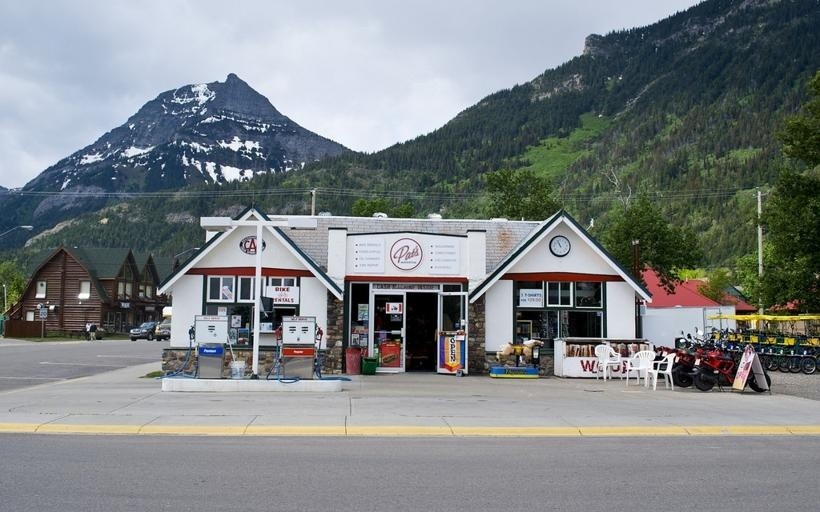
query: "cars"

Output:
[129,317,171,341]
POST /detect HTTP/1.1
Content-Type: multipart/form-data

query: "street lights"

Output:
[0,225,33,237]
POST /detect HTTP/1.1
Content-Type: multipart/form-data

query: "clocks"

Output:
[549,235,571,257]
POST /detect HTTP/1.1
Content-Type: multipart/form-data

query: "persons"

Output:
[85,322,92,340]
[89,321,97,342]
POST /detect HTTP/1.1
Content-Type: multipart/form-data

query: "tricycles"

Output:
[706,313,820,375]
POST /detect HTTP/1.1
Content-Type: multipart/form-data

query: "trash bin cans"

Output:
[346,347,377,375]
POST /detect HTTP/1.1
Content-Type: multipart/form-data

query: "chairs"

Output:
[595,344,677,391]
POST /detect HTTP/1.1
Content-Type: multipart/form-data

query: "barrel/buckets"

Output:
[230,361,246,379]
[345,348,362,375]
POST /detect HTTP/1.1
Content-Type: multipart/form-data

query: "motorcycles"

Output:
[654,326,771,393]
